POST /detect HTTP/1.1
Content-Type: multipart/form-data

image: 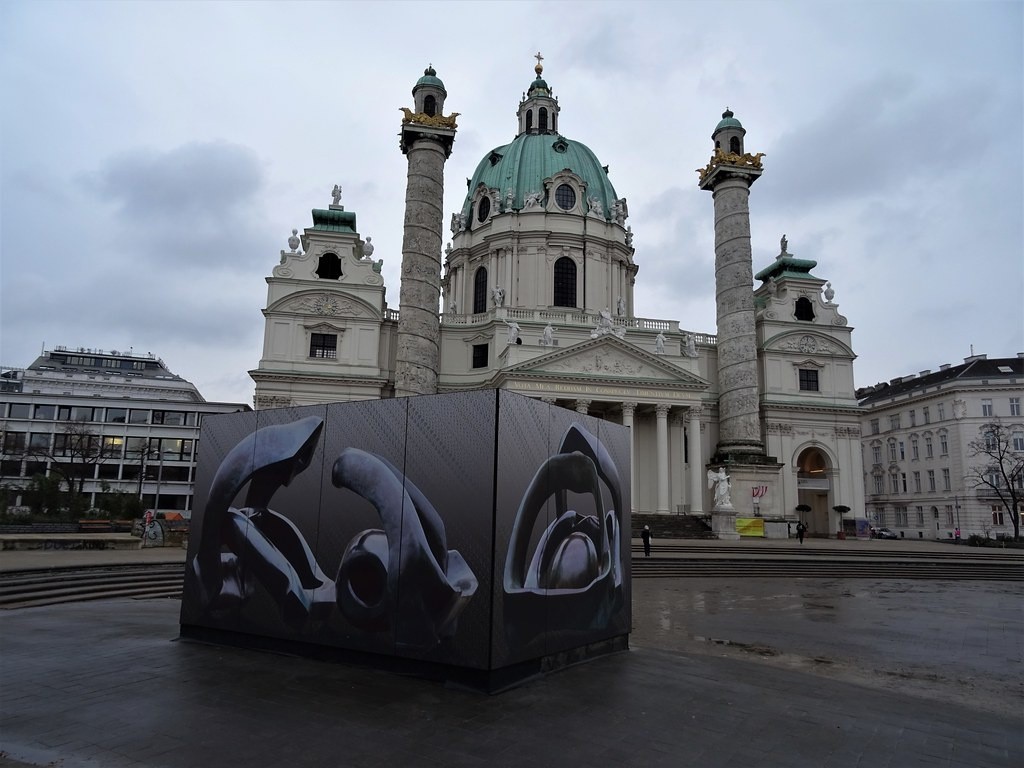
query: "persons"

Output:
[491,284,505,308]
[619,297,625,315]
[333,185,341,205]
[526,189,538,207]
[448,297,457,314]
[503,317,522,343]
[787,520,806,544]
[544,322,558,345]
[714,467,732,505]
[610,199,626,225]
[781,234,788,251]
[688,331,698,356]
[592,196,604,217]
[954,528,960,539]
[505,188,515,210]
[494,190,502,212]
[597,307,613,333]
[654,331,666,354]
[640,525,654,558]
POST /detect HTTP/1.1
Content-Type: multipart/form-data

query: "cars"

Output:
[872,528,897,539]
